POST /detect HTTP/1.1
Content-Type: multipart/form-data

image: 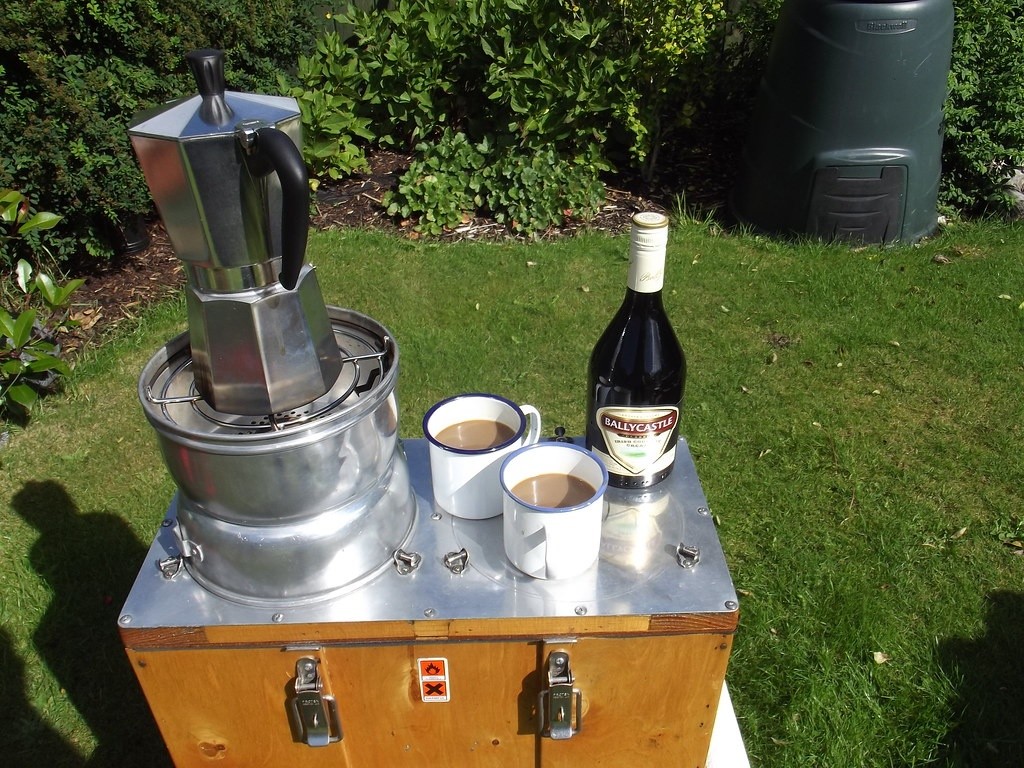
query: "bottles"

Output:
[585,212,687,489]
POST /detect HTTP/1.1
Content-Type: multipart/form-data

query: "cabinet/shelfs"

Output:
[117,437,741,768]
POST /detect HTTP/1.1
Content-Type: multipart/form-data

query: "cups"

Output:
[499,442,609,580]
[423,393,541,519]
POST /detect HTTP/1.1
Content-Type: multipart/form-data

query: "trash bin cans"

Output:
[728,0,955,244]
